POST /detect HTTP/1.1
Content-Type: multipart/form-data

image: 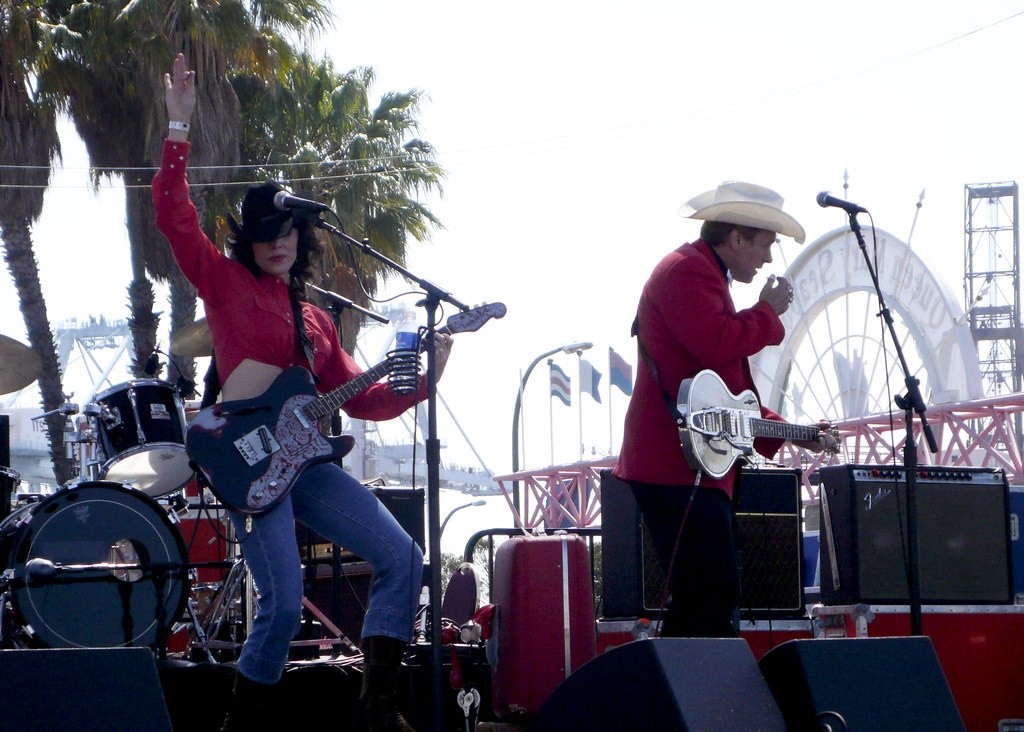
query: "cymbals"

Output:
[0,334,44,396]
[168,315,215,358]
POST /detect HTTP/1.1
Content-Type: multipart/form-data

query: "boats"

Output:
[462,467,512,497]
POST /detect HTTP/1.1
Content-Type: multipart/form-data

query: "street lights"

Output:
[512,341,593,527]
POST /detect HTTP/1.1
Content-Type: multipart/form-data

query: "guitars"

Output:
[677,368,841,479]
[187,302,507,513]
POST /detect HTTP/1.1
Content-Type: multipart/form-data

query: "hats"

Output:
[226,179,315,242]
[681,180,805,245]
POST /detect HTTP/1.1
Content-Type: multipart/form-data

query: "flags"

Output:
[610,347,632,396]
[550,364,571,407]
[579,358,602,404]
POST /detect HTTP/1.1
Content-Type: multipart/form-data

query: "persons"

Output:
[610,180,837,638]
[150,53,454,732]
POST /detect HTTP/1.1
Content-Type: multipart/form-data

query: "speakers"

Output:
[599,468,806,620]
[757,636,967,732]
[817,463,1014,607]
[525,637,787,732]
[285,483,427,653]
[0,648,173,732]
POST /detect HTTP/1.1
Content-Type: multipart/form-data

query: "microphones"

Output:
[145,350,159,375]
[817,191,868,213]
[274,190,330,212]
[25,558,57,585]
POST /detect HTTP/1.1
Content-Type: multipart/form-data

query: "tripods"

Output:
[185,529,370,670]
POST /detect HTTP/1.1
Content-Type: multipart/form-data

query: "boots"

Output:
[220,668,272,732]
[359,636,419,732]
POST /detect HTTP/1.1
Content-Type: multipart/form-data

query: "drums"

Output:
[88,376,198,501]
[0,467,193,650]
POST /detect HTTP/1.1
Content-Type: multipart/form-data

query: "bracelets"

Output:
[168,120,190,132]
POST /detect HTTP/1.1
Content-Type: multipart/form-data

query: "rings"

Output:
[788,288,794,303]
[767,275,776,282]
[438,348,443,352]
[824,448,832,453]
